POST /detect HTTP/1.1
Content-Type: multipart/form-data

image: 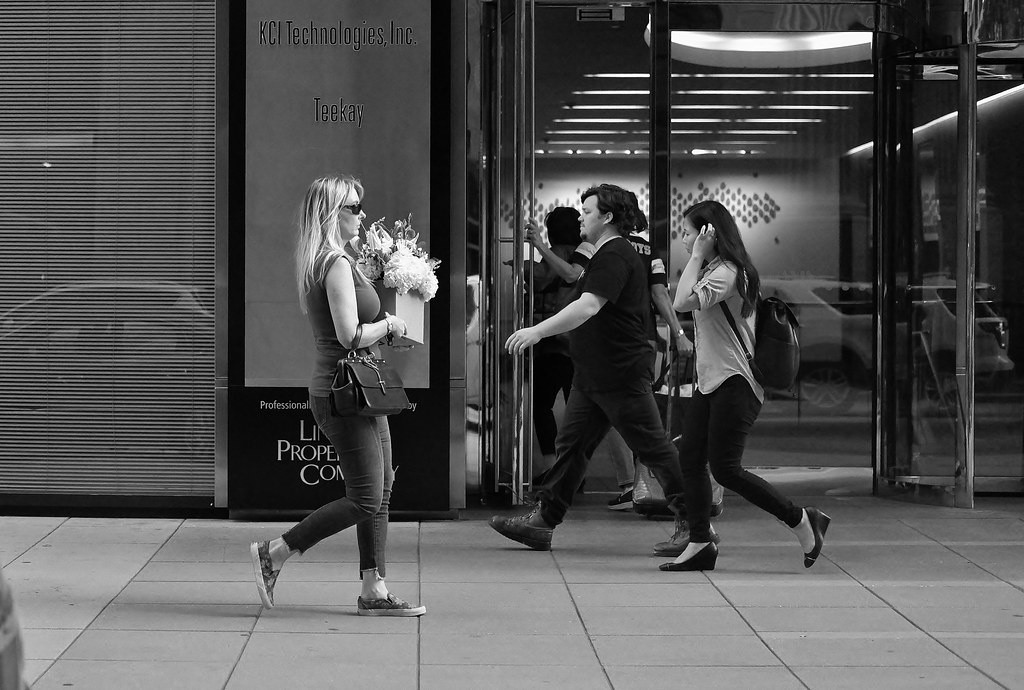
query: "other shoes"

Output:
[578,477,586,491]
[532,469,551,488]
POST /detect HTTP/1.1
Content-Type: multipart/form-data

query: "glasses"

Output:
[339,204,361,215]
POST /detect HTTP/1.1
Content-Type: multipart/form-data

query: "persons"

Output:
[489,184,832,572]
[251,173,426,617]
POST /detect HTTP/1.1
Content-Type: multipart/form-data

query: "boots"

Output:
[489,500,552,550]
[654,492,720,557]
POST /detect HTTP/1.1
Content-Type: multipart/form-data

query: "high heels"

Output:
[659,542,718,571]
[805,507,831,568]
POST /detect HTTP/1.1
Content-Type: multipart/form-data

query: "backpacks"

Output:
[710,259,799,391]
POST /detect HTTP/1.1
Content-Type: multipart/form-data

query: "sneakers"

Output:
[608,490,633,510]
[251,541,280,610]
[357,592,426,616]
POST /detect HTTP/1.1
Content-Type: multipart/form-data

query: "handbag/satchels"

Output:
[330,325,410,417]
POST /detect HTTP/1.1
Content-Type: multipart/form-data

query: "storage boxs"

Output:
[374,280,424,347]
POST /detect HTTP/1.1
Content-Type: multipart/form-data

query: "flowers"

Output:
[349,213,442,302]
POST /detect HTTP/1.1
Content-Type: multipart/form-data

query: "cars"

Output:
[759,271,1015,415]
[0,275,217,410]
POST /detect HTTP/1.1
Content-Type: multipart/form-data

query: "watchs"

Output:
[673,328,684,339]
[384,318,393,336]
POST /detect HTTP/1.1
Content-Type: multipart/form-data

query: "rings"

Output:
[710,234,714,238]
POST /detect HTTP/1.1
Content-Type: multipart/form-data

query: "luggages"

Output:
[633,345,724,519]
